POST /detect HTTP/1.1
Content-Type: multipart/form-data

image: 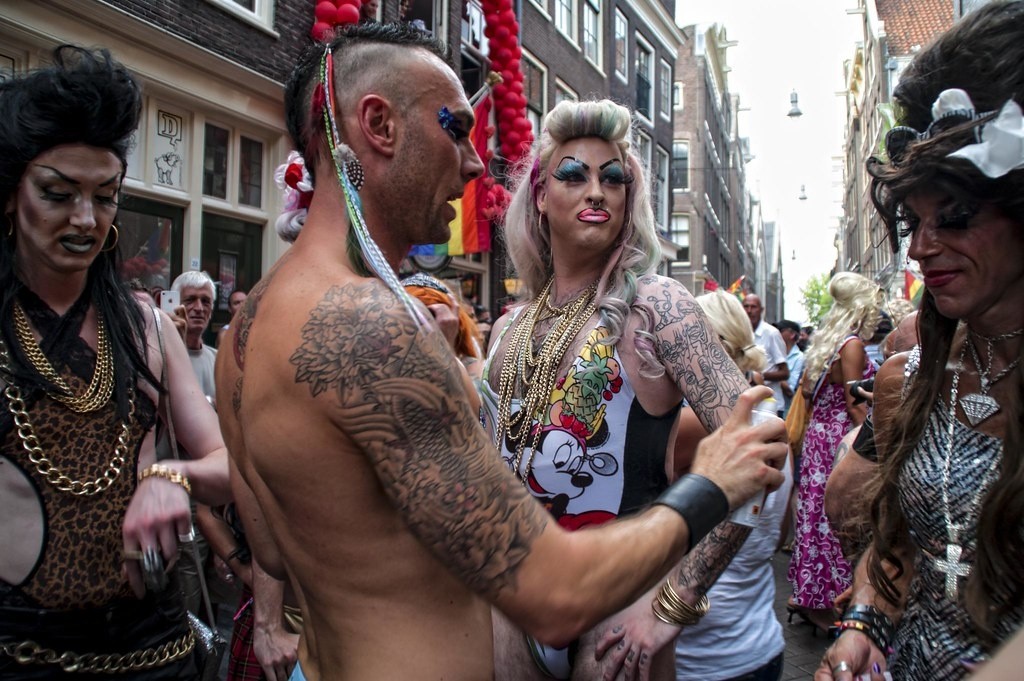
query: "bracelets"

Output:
[840,612,891,644]
[835,621,887,651]
[844,604,895,636]
[140,465,191,491]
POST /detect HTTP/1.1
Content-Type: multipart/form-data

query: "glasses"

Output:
[885,109,1002,167]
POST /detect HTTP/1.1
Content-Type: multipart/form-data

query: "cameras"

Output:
[847,370,875,406]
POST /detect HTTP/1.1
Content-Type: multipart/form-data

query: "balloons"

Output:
[480,0,533,223]
[311,1,362,43]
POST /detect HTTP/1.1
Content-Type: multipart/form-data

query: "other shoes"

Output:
[781,538,794,556]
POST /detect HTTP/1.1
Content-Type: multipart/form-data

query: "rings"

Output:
[831,661,853,676]
[140,548,164,575]
[119,550,142,560]
[178,525,196,542]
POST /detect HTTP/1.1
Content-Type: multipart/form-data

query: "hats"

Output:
[772,319,800,334]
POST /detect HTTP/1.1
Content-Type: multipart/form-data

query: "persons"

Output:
[213,34,790,681]
[810,3,1023,681]
[2,38,232,681]
[476,99,755,681]
[647,472,729,554]
[1,266,1024,681]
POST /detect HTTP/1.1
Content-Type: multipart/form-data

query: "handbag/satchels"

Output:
[784,368,810,456]
[186,610,228,681]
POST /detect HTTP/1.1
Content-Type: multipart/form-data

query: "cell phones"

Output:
[161,291,180,316]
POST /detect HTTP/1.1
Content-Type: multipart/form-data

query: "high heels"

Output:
[805,606,841,637]
[785,604,810,623]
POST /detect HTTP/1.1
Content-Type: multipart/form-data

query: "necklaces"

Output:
[967,323,1024,395]
[934,328,1005,599]
